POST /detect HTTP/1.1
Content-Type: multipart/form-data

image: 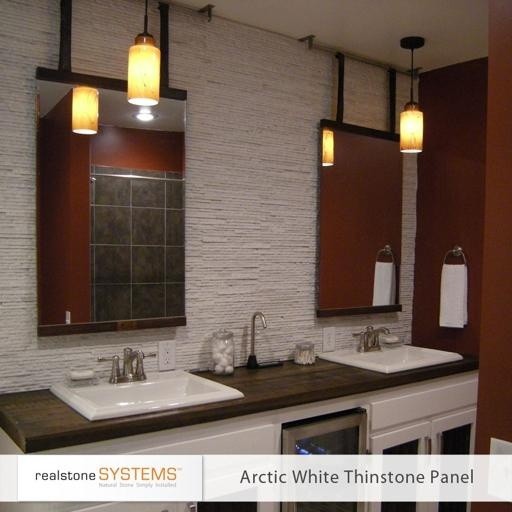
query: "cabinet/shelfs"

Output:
[367,373,480,512]
[0,422,279,512]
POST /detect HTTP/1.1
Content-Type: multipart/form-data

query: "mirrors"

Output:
[316,114,402,319]
[33,65,186,336]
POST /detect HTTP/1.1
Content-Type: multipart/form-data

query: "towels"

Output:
[440,264,468,329]
[372,262,397,306]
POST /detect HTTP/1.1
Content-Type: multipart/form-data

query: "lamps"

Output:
[70,84,100,135]
[322,127,335,167]
[126,0,160,107]
[399,36,425,152]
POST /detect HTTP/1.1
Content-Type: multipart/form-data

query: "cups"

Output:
[291,342,318,365]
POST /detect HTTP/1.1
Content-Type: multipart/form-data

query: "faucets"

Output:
[246,310,268,369]
[365,326,390,350]
[123,346,144,380]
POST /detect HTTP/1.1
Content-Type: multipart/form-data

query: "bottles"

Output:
[212,327,236,374]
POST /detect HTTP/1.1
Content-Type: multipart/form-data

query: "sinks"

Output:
[318,343,464,375]
[50,370,246,422]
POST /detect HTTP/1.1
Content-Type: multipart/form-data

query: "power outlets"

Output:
[157,339,176,371]
